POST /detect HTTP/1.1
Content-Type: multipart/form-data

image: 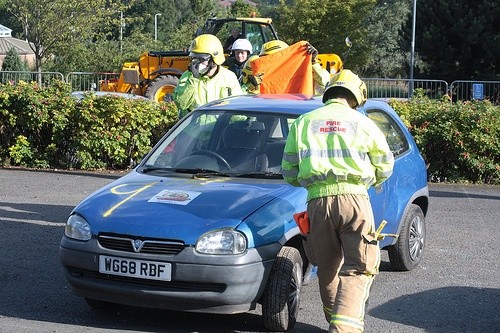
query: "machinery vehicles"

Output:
[95,17,345,107]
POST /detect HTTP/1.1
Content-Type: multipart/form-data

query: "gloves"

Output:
[189,58,200,78]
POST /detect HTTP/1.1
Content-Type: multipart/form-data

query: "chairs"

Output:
[268,141,285,168]
[210,120,268,173]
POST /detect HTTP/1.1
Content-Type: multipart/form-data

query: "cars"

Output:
[59,92,431,331]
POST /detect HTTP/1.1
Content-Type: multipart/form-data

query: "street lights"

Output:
[154,13,163,51]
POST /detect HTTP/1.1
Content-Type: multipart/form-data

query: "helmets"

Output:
[321,70,367,107]
[242,55,261,83]
[187,35,226,65]
[259,39,288,55]
[232,39,253,55]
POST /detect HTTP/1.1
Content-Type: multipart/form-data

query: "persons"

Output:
[224,28,331,98]
[172,34,256,165]
[280,69,395,333]
